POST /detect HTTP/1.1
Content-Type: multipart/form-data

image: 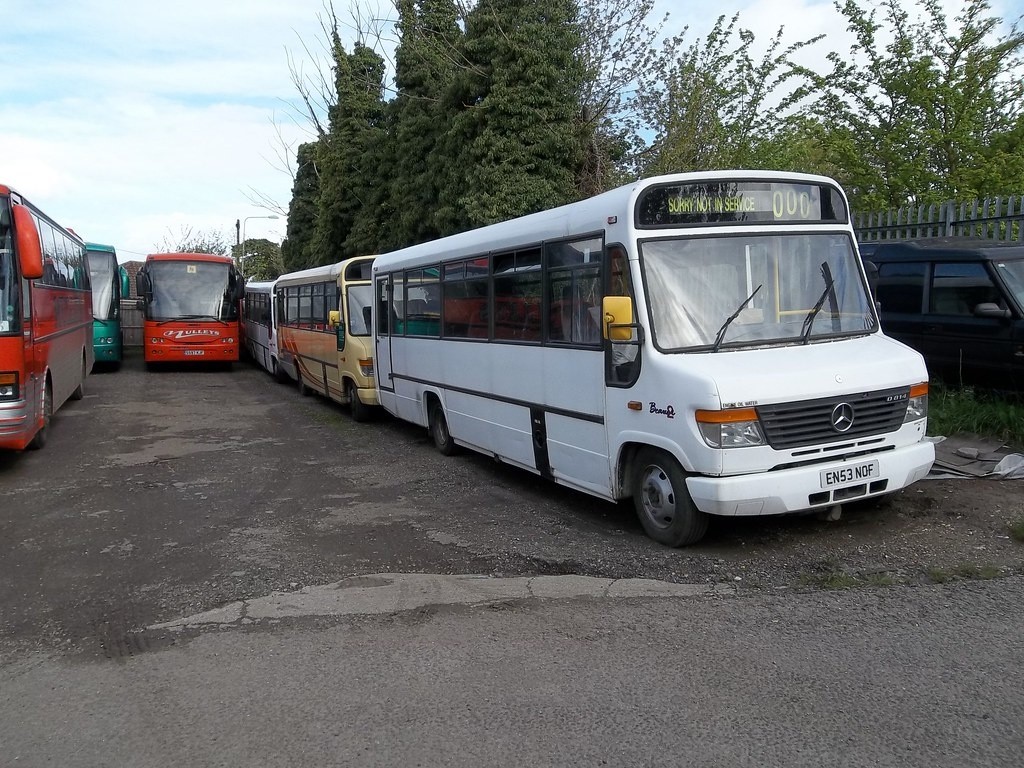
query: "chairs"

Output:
[446,297,596,343]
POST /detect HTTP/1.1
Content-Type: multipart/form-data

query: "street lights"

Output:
[242,214,279,276]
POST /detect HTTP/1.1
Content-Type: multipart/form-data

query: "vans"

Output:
[809,238,1024,403]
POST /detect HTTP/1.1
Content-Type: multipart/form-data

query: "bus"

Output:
[275,254,376,410]
[245,283,278,377]
[135,254,246,362]
[386,269,441,335]
[0,184,94,452]
[368,169,935,547]
[444,244,585,339]
[85,243,129,368]
[42,258,75,288]
